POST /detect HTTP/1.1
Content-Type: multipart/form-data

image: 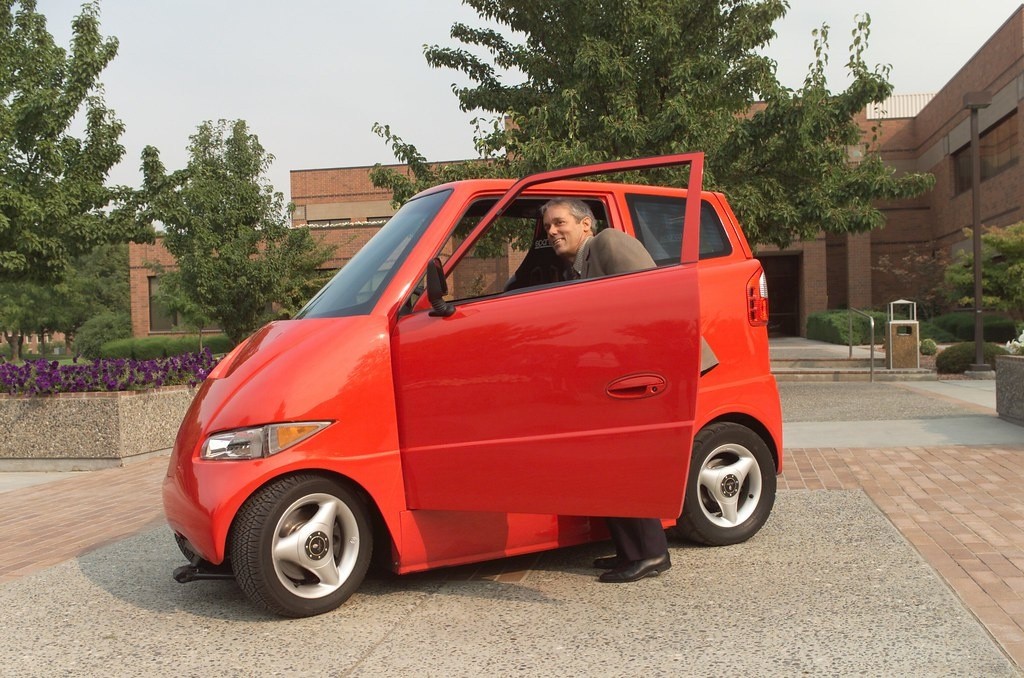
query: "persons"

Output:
[540,196,672,583]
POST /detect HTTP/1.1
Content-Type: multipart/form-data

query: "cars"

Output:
[160,158,783,615]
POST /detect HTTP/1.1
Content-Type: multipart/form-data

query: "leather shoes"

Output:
[594,554,671,583]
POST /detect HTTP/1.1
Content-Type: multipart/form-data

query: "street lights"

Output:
[961,91,994,365]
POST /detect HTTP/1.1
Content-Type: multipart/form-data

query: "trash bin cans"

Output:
[884,297,923,371]
